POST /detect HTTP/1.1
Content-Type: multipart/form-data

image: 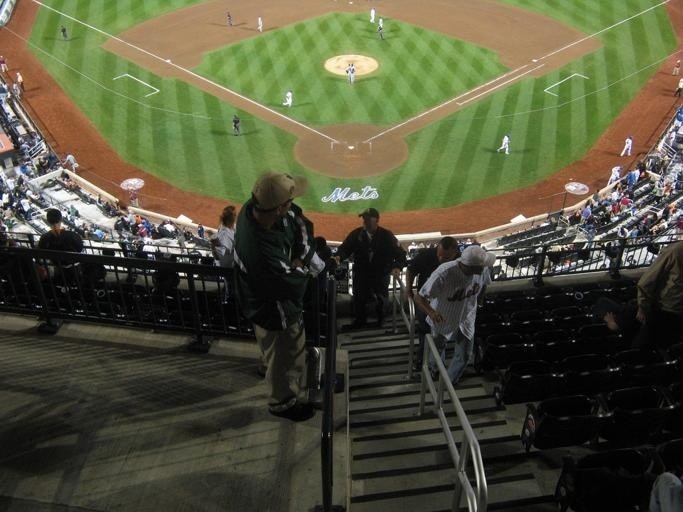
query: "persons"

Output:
[337,208,407,327]
[406,237,460,369]
[60,24,67,40]
[257,16,264,32]
[284,90,293,107]
[346,64,356,84]
[565,106,682,262]
[226,11,232,27]
[415,244,495,393]
[497,133,510,154]
[672,60,680,75]
[232,167,326,423]
[673,79,682,97]
[369,7,384,39]
[600,239,682,351]
[0,54,246,320]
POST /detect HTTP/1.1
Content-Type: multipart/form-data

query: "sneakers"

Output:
[269,402,316,422]
[255,367,268,378]
[431,369,440,381]
[411,361,423,372]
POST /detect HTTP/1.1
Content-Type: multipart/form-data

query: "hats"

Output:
[461,243,496,267]
[250,169,309,209]
[359,207,380,219]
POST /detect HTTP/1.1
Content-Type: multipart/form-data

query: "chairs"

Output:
[401,104,683,510]
[0,71,349,342]
